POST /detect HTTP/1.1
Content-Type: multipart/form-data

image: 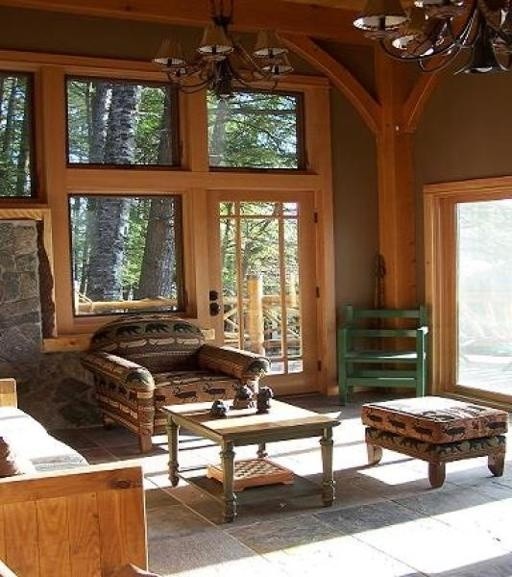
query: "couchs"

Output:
[0,377,149,577]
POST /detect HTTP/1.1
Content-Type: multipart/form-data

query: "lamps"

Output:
[154,0,293,103]
[353,0,512,77]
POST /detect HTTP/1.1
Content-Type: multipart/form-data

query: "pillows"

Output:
[0,433,25,479]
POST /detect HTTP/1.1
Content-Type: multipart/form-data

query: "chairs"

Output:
[80,311,274,454]
[336,303,429,406]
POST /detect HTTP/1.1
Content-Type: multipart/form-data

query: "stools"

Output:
[362,396,507,487]
